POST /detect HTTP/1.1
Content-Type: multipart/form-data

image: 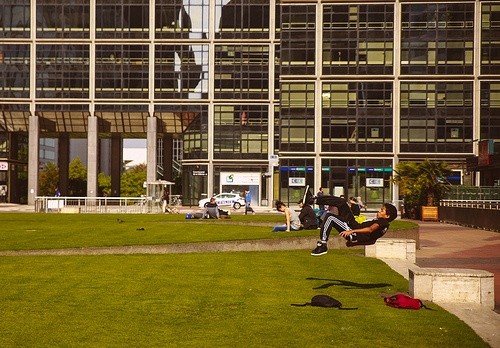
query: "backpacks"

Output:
[384,294,436,311]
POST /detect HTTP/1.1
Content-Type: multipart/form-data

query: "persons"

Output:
[206,197,228,216]
[272,200,301,232]
[297,199,317,230]
[161,187,172,215]
[1,186,8,204]
[317,187,325,211]
[54,186,61,197]
[243,188,255,216]
[348,196,367,217]
[303,185,397,256]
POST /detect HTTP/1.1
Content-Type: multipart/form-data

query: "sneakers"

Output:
[311,241,327,256]
[303,185,315,205]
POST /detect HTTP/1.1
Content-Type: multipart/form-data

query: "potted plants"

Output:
[383,160,453,222]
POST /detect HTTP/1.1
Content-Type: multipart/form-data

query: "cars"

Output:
[199,192,246,209]
